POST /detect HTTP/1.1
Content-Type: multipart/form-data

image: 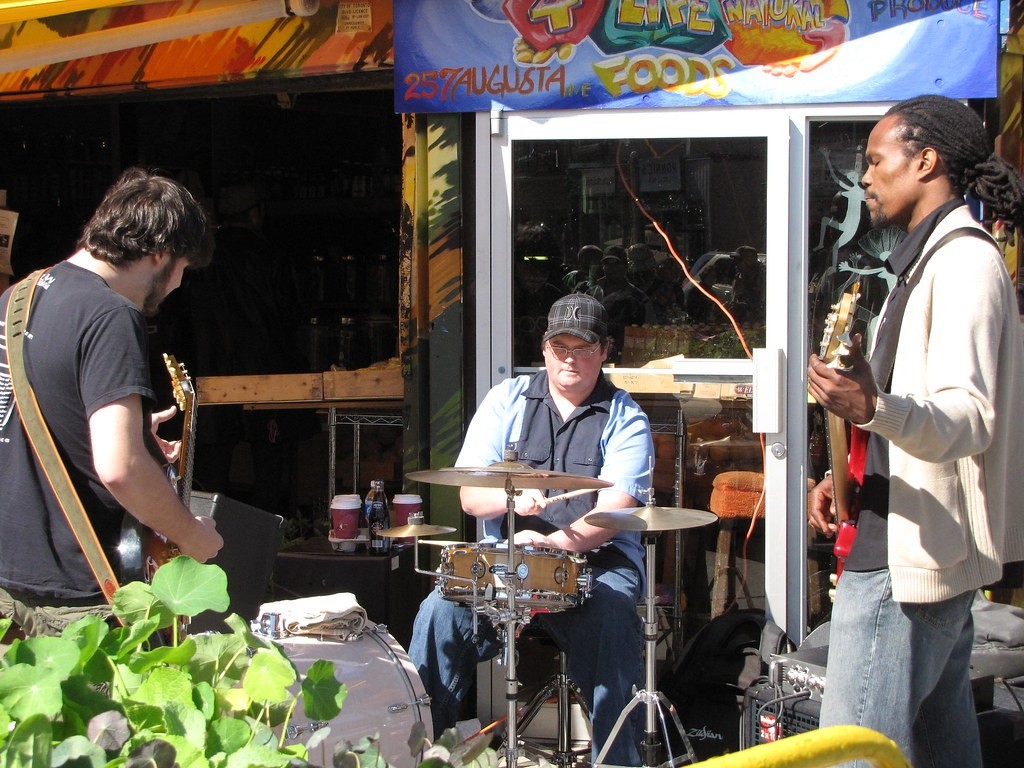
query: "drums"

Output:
[242,596,435,768]
[433,537,594,617]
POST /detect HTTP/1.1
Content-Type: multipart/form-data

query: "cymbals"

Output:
[401,462,616,490]
[374,520,463,542]
[581,510,719,532]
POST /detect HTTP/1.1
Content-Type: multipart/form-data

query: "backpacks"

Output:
[656,608,800,768]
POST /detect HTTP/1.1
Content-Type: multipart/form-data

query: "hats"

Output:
[729,245,757,261]
[543,291,609,344]
[601,245,629,268]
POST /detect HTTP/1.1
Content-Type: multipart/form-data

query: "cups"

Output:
[331,494,362,539]
[392,494,423,545]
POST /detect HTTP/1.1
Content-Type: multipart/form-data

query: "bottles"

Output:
[365,481,388,526]
[368,481,390,555]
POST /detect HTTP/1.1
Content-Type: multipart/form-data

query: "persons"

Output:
[562,244,766,368]
[410,291,656,768]
[0,167,224,636]
[803,96,1024,768]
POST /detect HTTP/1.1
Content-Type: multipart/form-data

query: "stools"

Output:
[488,616,593,768]
[707,470,824,621]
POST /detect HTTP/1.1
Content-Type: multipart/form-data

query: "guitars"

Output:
[136,345,207,661]
[803,276,873,608]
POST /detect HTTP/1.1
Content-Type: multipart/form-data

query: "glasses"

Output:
[546,342,602,360]
